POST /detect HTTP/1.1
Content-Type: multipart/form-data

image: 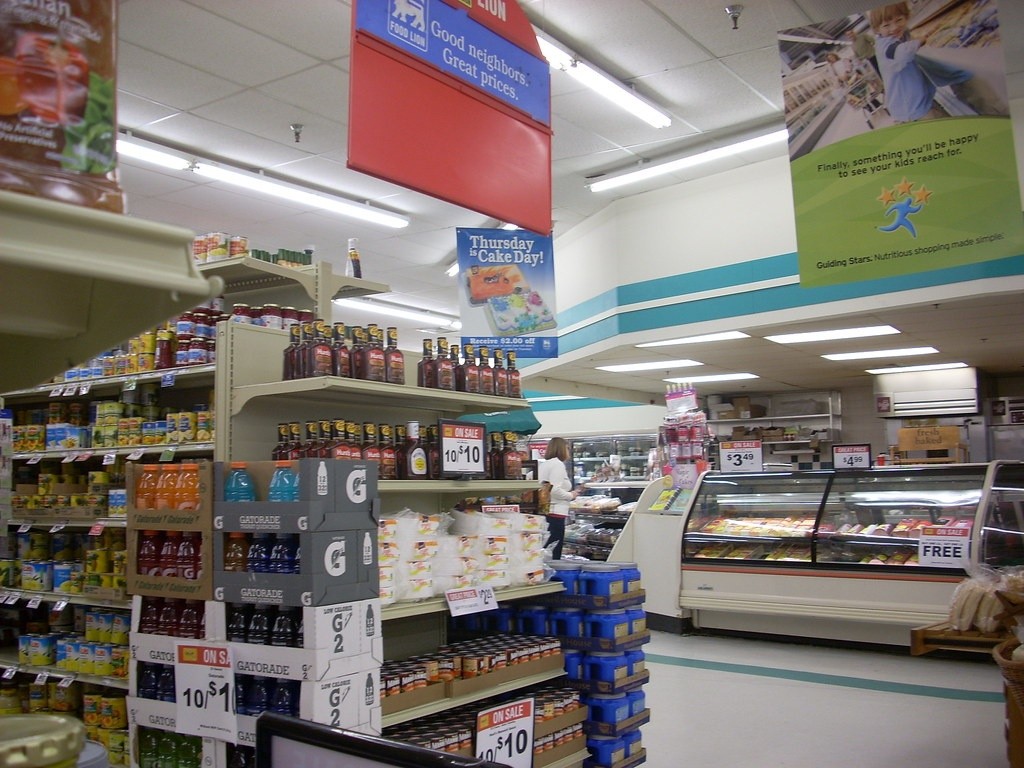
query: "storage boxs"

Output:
[225,597,387,682]
[126,526,213,601]
[124,457,213,528]
[128,722,216,768]
[717,409,736,419]
[211,459,381,532]
[235,668,384,748]
[212,529,379,607]
[735,404,766,418]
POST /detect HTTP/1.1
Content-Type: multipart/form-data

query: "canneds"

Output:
[380,634,583,755]
[61,294,313,382]
[192,232,247,264]
[0,387,215,768]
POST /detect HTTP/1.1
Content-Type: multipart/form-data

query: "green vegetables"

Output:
[61,74,119,176]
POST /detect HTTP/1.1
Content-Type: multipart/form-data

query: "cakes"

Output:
[465,264,555,335]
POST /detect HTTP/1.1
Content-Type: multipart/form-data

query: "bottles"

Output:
[366,604,374,636]
[362,531,373,565]
[344,237,362,280]
[281,319,405,385]
[365,673,374,705]
[415,336,523,399]
[134,421,520,768]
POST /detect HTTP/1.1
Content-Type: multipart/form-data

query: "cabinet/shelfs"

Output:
[0,260,569,768]
[519,392,845,565]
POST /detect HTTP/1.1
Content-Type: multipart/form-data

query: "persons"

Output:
[826,0,950,124]
[539,436,576,559]
